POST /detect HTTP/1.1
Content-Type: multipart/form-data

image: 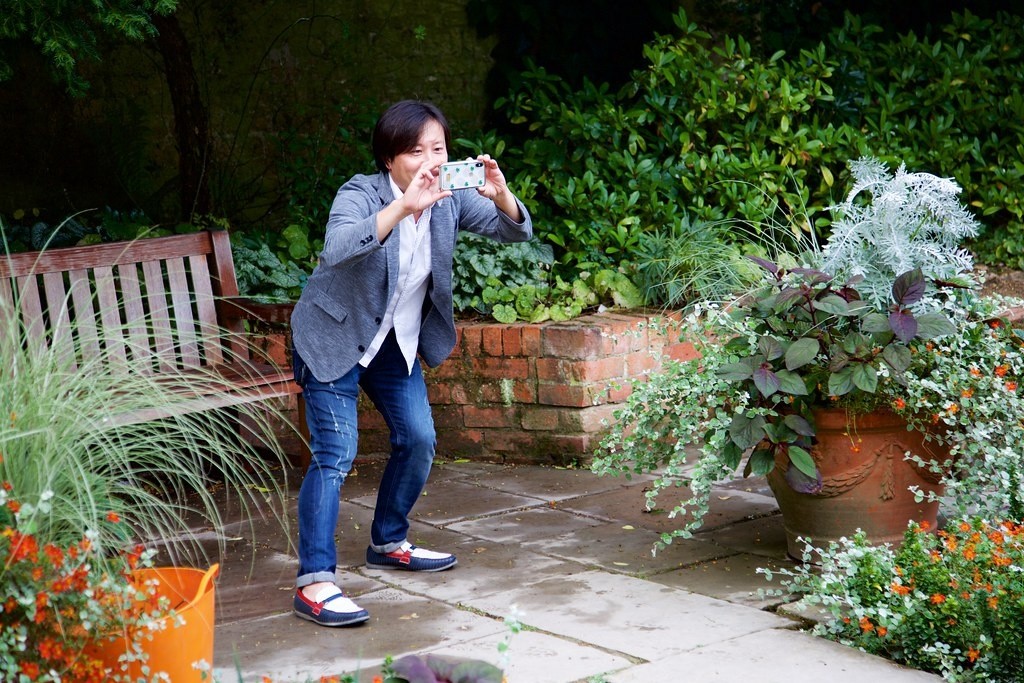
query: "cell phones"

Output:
[438,159,486,191]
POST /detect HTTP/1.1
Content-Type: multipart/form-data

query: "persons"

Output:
[288,99,533,628]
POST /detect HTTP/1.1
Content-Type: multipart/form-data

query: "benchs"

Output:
[0,228,312,482]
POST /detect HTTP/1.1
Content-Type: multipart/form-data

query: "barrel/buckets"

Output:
[62,563,222,682]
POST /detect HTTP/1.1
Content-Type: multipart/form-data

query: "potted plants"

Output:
[591,153,1024,556]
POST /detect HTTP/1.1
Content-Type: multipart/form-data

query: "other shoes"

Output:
[294,587,370,627]
[365,542,459,572]
